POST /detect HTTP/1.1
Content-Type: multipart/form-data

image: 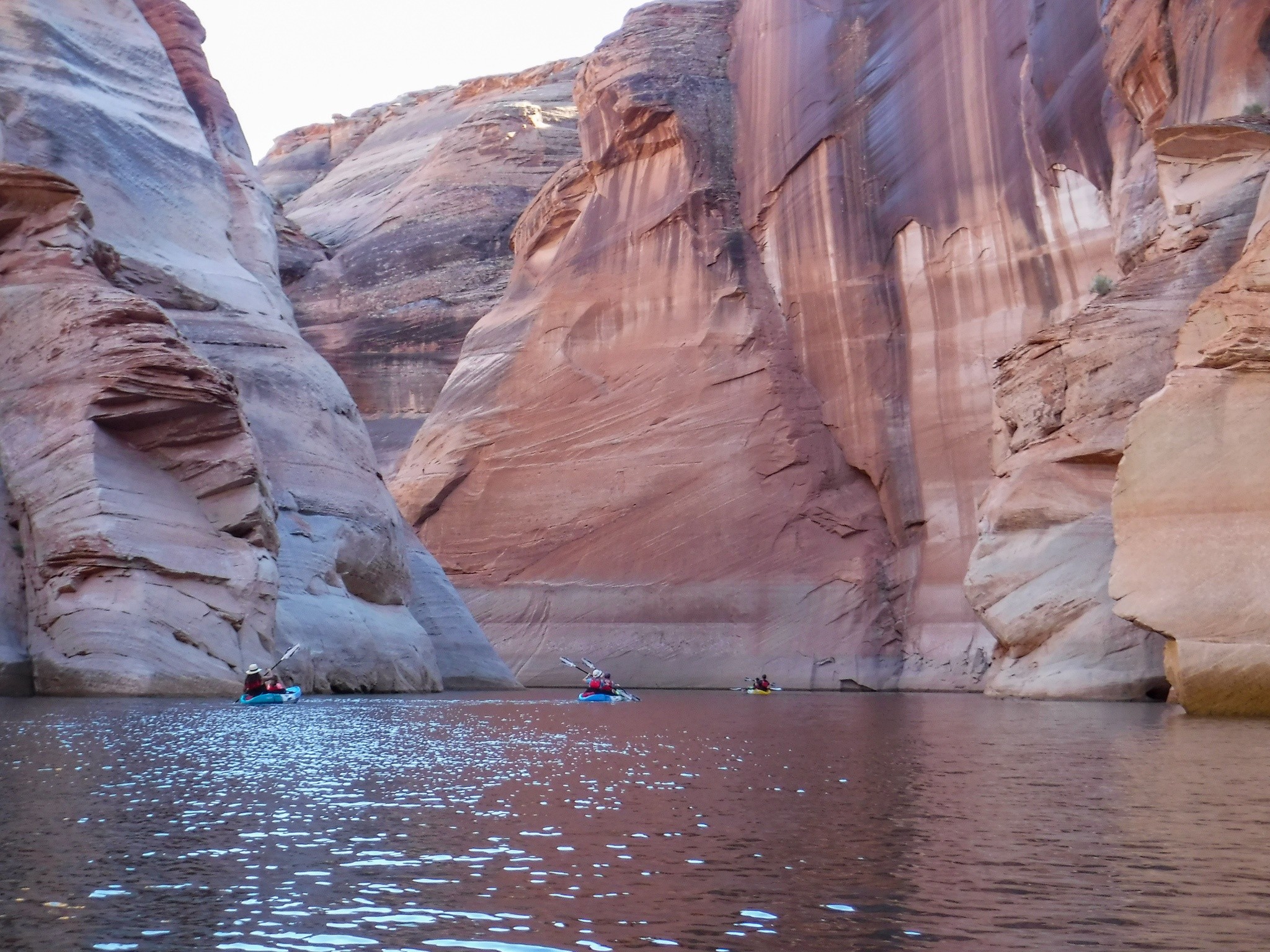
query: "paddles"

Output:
[744,677,776,687]
[581,658,641,701]
[233,644,300,704]
[559,656,632,701]
[730,688,781,690]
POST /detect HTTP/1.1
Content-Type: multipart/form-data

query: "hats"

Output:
[593,669,602,678]
[245,664,262,674]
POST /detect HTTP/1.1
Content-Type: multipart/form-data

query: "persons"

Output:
[243,664,286,696]
[583,668,617,694]
[746,674,772,692]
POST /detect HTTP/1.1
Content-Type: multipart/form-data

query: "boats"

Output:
[746,688,771,695]
[240,686,303,705]
[578,692,625,702]
